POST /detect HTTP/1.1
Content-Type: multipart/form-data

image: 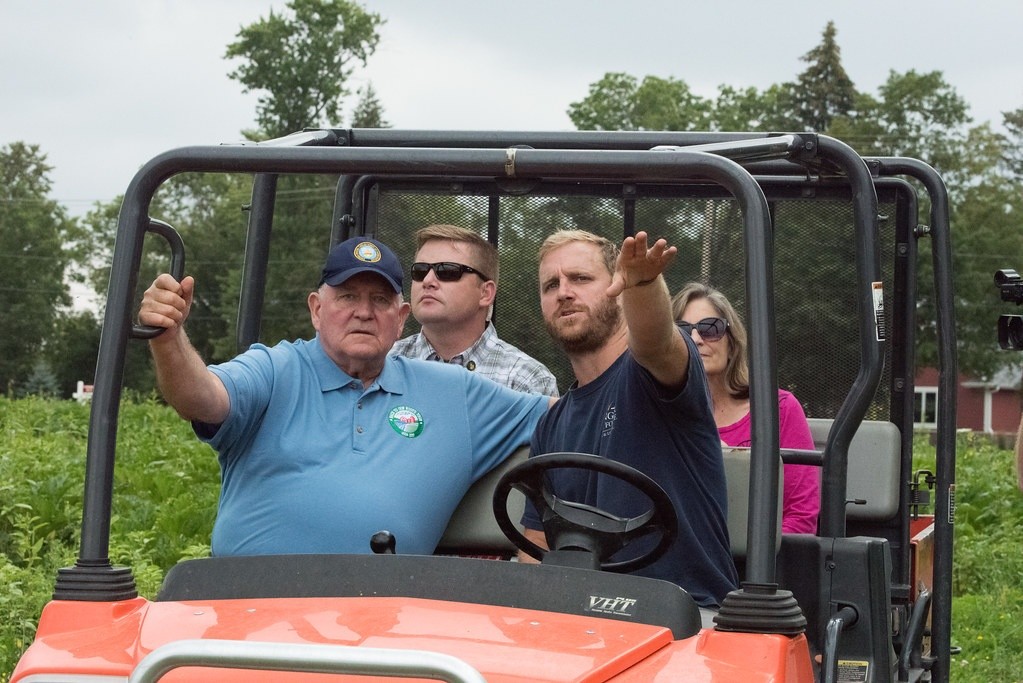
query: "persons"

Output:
[672,283,822,536]
[137,237,561,558]
[387,226,559,398]
[517,228,740,614]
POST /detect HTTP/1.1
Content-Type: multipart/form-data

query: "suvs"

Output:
[12,125,954,683]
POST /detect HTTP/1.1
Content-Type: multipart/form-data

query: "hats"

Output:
[318,236,406,296]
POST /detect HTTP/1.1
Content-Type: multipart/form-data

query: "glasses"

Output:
[410,261,491,283]
[676,318,730,342]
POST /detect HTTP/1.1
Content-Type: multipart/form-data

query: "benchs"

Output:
[804,416,902,538]
[434,446,783,618]
